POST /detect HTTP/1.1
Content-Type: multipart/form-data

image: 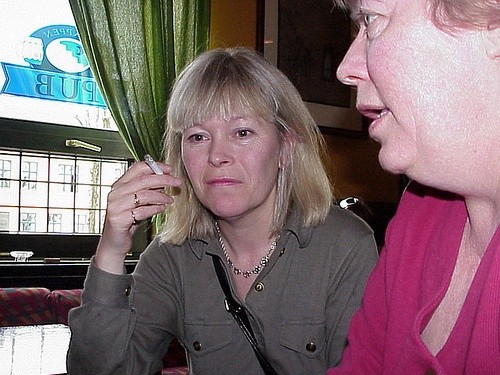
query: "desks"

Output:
[0,324,72,375]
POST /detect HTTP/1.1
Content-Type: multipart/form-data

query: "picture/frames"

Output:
[253,0,372,141]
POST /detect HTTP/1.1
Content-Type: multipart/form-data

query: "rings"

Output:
[131,209,138,224]
[134,193,141,207]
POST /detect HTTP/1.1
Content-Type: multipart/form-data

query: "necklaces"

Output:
[214,221,281,278]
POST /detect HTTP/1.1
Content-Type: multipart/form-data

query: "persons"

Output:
[325,0,500,375]
[66,48,380,375]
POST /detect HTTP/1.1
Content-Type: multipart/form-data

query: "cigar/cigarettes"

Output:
[143,154,164,176]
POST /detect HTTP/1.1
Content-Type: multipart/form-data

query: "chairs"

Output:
[48,289,82,328]
[161,337,189,375]
[0,287,52,325]
